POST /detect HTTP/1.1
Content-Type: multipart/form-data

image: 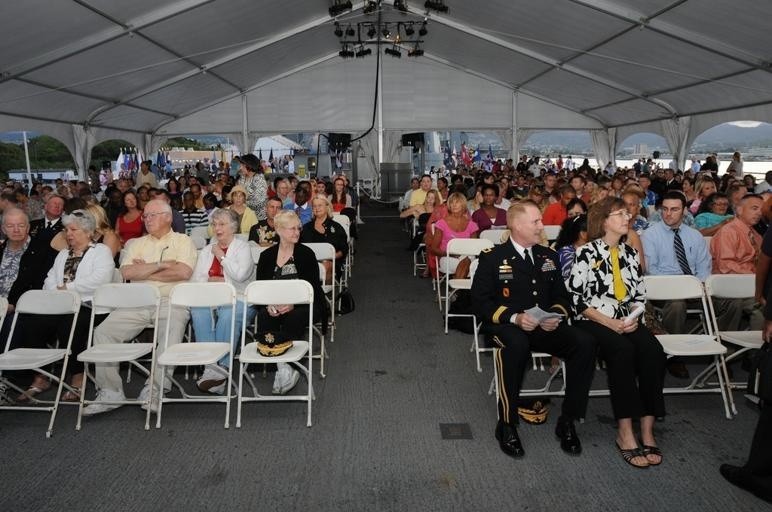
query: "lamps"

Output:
[328,0,449,58]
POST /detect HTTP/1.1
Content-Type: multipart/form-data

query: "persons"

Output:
[1,154,357,415]
[397,152,772,503]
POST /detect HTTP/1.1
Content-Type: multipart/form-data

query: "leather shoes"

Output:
[555,417,582,455]
[495,419,525,458]
[669,358,689,378]
[720,464,771,502]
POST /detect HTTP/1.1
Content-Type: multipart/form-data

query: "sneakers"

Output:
[421,272,428,279]
[447,291,456,302]
[197,364,227,395]
[138,383,162,413]
[81,388,128,417]
[271,365,300,395]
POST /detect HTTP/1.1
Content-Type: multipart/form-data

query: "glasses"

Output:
[609,212,632,218]
[141,211,167,220]
[70,211,87,219]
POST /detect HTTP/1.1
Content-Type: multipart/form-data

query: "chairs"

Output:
[235,280,315,429]
[412,204,767,419]
[2,290,82,437]
[76,283,162,430]
[0,210,355,378]
[156,282,237,430]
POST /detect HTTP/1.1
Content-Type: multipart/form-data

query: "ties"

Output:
[524,249,533,269]
[672,228,691,275]
[46,221,51,229]
[750,233,759,264]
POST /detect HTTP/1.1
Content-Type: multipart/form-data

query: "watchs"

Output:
[219,256,226,264]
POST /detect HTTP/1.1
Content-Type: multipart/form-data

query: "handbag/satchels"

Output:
[341,287,355,314]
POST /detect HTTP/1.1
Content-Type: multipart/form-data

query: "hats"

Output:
[253,331,296,357]
[517,396,552,425]
[236,154,260,170]
[227,185,248,201]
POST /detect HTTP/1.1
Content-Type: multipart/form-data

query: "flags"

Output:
[116,150,145,172]
[444,143,495,171]
[157,150,171,169]
[327,143,344,176]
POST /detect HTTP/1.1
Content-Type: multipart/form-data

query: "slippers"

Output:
[638,430,663,465]
[61,385,81,403]
[615,439,649,469]
[17,384,52,401]
